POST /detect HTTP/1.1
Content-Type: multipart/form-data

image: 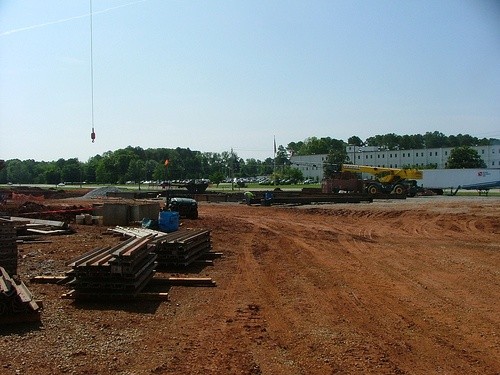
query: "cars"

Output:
[125,177,314,184]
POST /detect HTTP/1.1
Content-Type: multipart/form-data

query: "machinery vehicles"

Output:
[341,163,423,199]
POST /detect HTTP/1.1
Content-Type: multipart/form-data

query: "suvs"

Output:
[167,188,198,218]
